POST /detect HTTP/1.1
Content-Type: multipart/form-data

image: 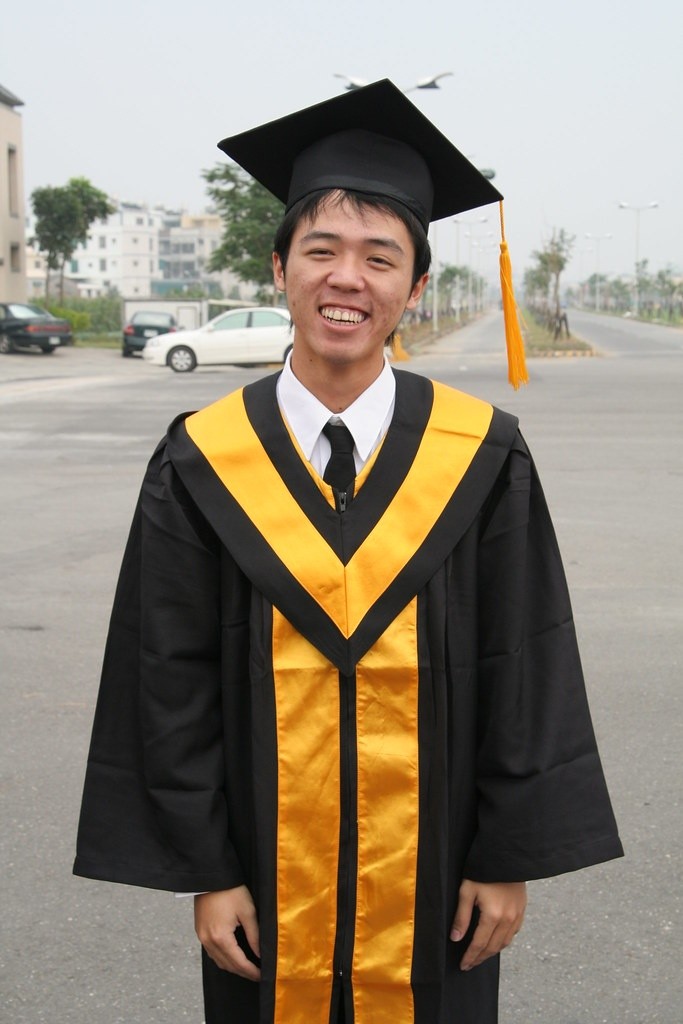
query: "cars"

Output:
[120,309,179,358]
[141,305,295,371]
[0,300,74,355]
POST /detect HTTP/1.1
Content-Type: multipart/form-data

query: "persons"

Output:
[73,79,624,1024]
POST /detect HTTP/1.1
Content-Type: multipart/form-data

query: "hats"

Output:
[216,78,530,391]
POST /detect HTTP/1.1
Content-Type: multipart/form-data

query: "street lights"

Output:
[451,218,489,318]
[618,200,662,313]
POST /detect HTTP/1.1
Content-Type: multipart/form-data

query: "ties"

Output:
[319,422,357,492]
